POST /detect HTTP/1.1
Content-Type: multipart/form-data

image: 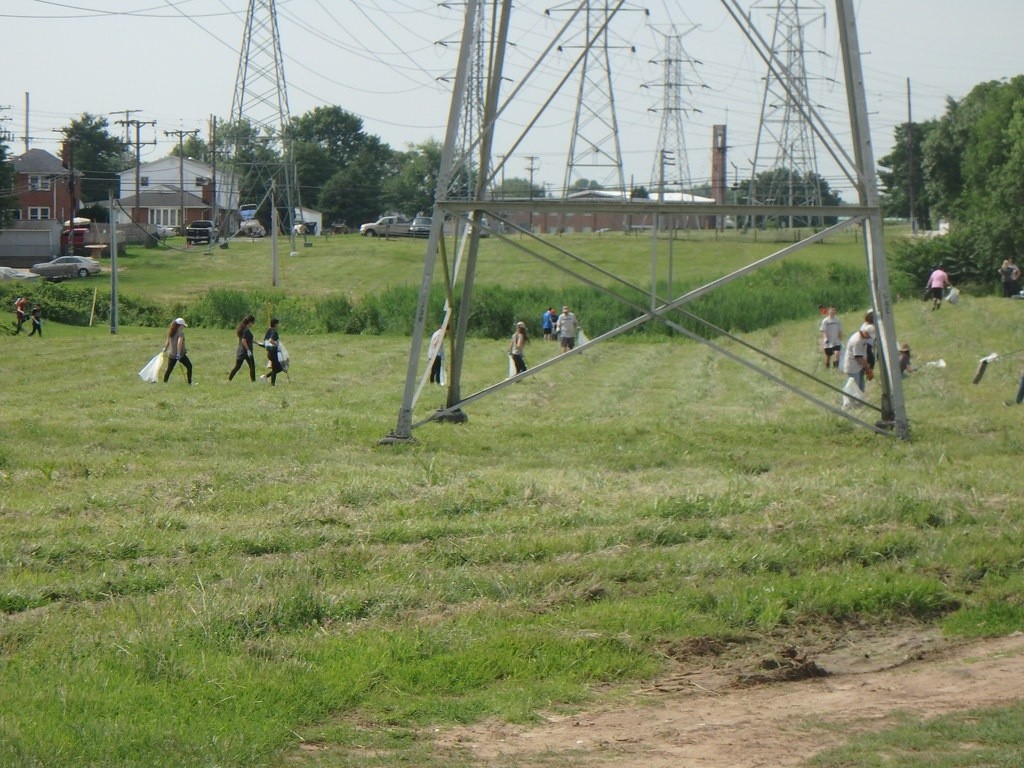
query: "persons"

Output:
[507,321,530,383]
[818,258,1024,405]
[15,297,42,337]
[428,324,450,384]
[228,316,280,386]
[163,318,198,385]
[543,305,581,352]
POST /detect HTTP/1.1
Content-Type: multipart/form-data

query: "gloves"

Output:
[823,336,831,349]
[247,350,252,360]
[176,353,181,360]
[556,328,561,334]
[576,326,581,331]
[865,368,874,381]
[163,348,167,353]
[35,321,40,324]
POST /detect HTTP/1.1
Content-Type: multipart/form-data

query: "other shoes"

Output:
[190,382,198,386]
[260,375,268,383]
[932,307,936,312]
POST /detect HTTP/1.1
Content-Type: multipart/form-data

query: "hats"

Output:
[899,342,912,352]
[563,306,568,313]
[175,318,187,327]
[1002,260,1008,268]
[516,322,528,330]
[551,309,555,314]
[865,325,875,339]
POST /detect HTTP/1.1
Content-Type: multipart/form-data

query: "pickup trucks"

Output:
[186,220,221,244]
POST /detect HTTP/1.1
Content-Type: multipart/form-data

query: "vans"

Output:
[62,228,87,247]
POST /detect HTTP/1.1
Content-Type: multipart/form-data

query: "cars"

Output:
[467,218,492,238]
[157,225,178,240]
[360,216,412,237]
[238,204,258,220]
[30,255,101,279]
[408,216,433,237]
